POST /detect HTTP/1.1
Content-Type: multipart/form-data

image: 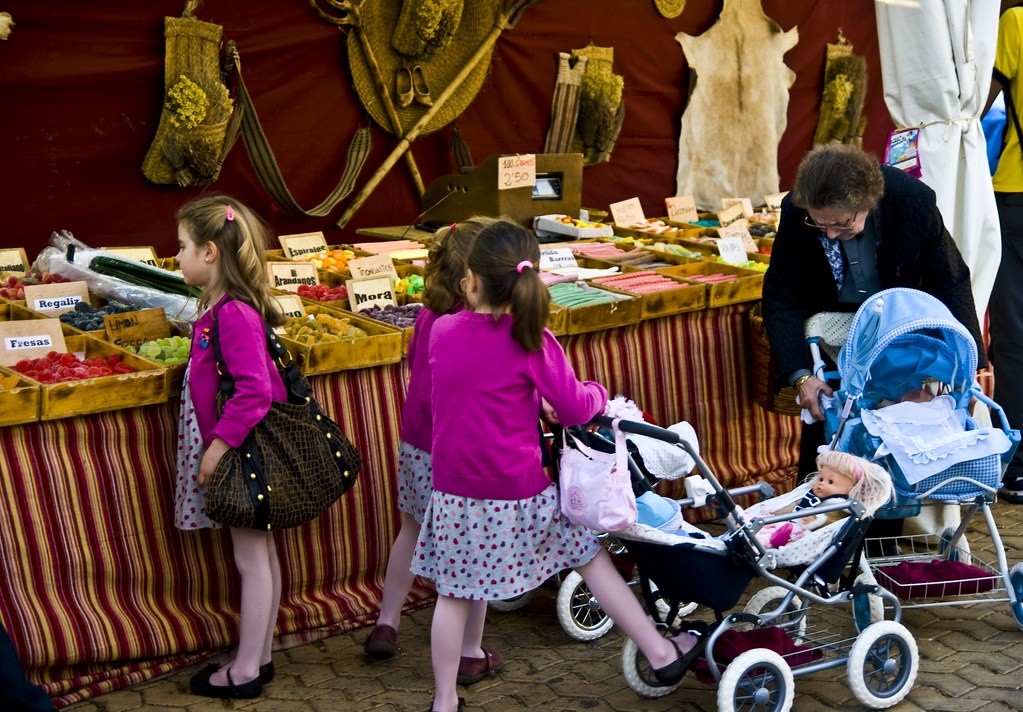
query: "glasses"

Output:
[804,214,860,231]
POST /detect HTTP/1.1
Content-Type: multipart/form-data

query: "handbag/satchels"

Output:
[559,418,637,530]
[205,295,363,528]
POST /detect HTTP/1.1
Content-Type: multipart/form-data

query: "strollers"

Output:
[805,288,1023,636]
[486,397,920,712]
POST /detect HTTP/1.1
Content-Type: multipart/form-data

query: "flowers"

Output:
[822,73,855,115]
[581,68,624,166]
[165,73,236,127]
[414,0,464,55]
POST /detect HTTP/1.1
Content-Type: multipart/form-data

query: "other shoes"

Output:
[365,624,397,657]
[190,667,262,699]
[456,649,502,685]
[425,698,466,712]
[648,621,711,687]
[999,465,1022,502]
[198,661,276,684]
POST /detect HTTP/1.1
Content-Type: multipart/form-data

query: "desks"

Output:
[0,193,993,697]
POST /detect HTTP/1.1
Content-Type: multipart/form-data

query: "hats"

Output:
[637,489,683,535]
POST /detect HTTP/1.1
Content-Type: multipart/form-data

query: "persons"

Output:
[762,146,988,563]
[982,0,1023,502]
[173,195,288,701]
[363,215,502,684]
[901,389,934,403]
[732,451,893,550]
[409,220,707,712]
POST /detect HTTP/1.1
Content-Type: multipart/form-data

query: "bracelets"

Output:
[796,373,811,391]
